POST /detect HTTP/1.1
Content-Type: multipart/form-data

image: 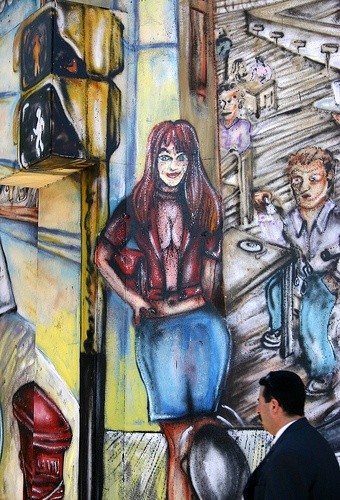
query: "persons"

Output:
[240,370,340,500]
